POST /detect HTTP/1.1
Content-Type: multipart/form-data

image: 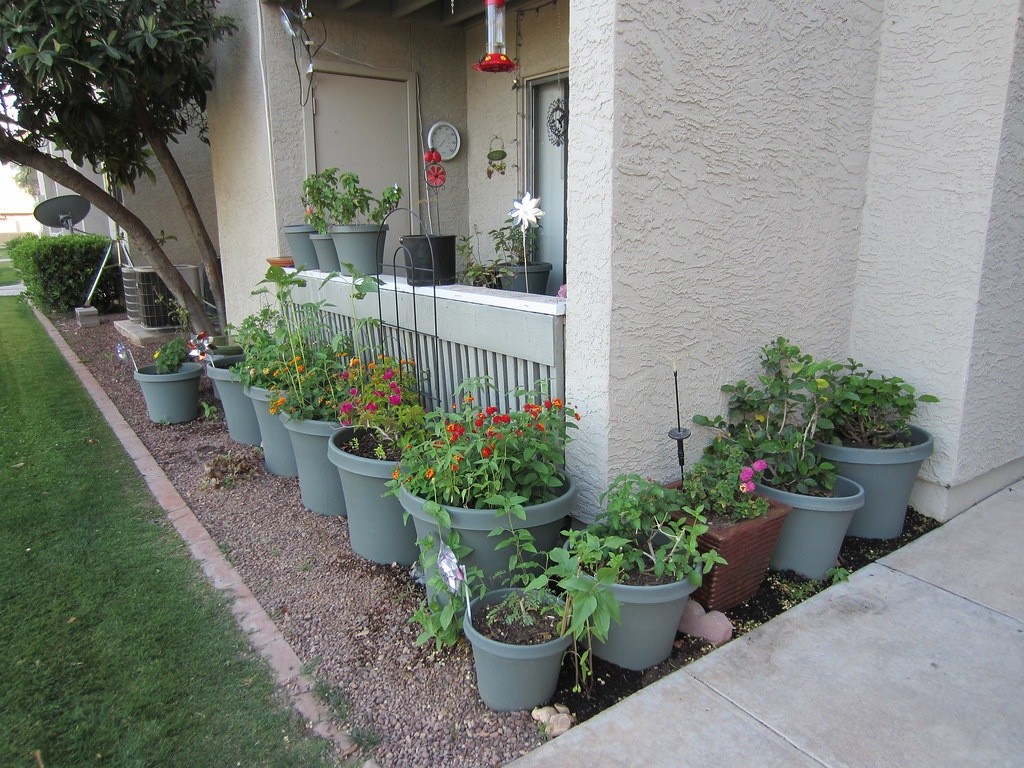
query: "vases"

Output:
[133,361,203,426]
[245,385,297,476]
[277,410,576,628]
[206,357,264,446]
[657,479,790,612]
[205,352,223,399]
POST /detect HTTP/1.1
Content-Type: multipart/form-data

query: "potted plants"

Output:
[460,337,943,712]
[283,166,553,293]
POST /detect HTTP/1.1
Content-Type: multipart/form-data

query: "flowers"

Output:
[683,436,774,520]
[154,263,580,516]
[508,192,545,294]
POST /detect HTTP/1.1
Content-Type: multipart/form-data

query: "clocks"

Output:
[428,121,461,161]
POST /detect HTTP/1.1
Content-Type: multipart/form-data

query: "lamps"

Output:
[477,0,516,72]
[279,0,314,38]
